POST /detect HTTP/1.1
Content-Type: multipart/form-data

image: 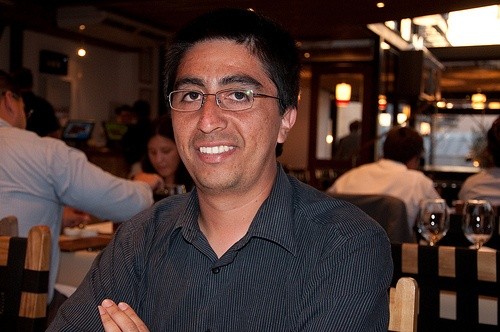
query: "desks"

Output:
[59,217,121,251]
[420,164,483,204]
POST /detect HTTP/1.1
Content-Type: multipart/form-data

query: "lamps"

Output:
[335,82,351,101]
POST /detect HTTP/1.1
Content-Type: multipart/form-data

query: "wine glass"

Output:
[464,199,494,251]
[418,200,450,247]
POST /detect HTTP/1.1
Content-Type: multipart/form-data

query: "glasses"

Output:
[165,87,278,112]
[14,94,34,119]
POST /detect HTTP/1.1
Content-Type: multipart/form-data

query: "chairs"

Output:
[310,154,356,195]
[332,192,411,294]
[386,277,420,332]
[0,214,53,332]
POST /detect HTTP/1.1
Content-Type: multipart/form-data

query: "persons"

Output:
[15,66,63,139]
[459,114,500,215]
[45,6,396,332]
[122,98,156,160]
[100,104,133,151]
[127,117,195,204]
[331,120,366,179]
[324,127,448,248]
[1,56,166,323]
